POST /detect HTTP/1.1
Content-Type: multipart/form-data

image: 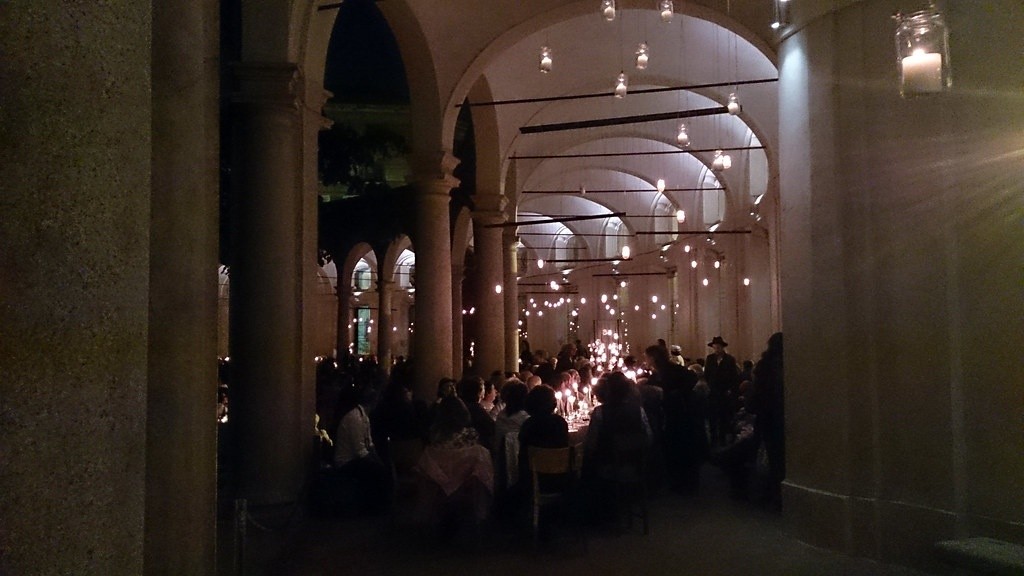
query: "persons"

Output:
[298,324,797,519]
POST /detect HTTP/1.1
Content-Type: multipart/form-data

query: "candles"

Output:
[529,364,646,428]
[322,1,956,356]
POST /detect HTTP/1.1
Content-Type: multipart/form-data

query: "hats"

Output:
[707,337,731,349]
